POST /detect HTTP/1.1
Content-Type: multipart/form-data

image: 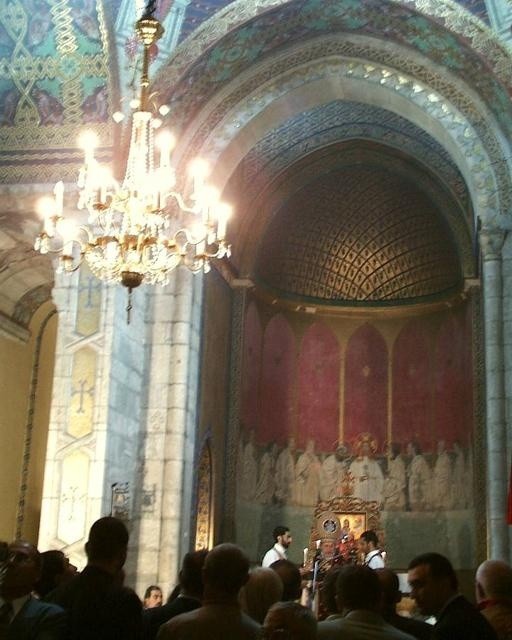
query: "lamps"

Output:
[33,1,234,326]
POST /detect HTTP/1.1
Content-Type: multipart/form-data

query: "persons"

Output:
[240,432,472,514]
[1,517,512,639]
